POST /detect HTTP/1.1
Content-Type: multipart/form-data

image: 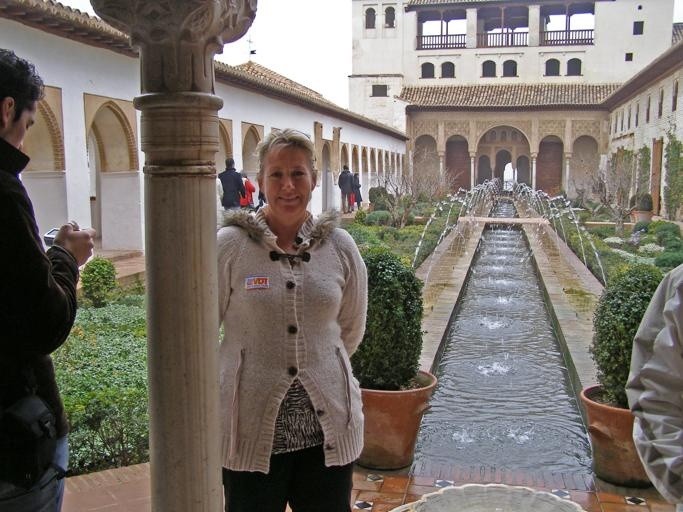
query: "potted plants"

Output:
[634,191,653,224]
[349,246,438,469]
[628,194,639,223]
[580,263,665,489]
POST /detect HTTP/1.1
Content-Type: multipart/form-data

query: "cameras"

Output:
[44,228,81,247]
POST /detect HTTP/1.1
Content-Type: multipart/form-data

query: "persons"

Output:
[218,158,255,210]
[216,129,368,512]
[625,263,683,512]
[0,49,96,512]
[338,164,362,214]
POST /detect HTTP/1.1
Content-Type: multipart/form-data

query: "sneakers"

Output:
[344,207,361,214]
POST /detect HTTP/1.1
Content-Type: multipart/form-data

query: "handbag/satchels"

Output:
[350,193,355,206]
[0,392,57,489]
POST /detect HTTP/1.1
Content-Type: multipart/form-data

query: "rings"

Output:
[67,222,77,231]
